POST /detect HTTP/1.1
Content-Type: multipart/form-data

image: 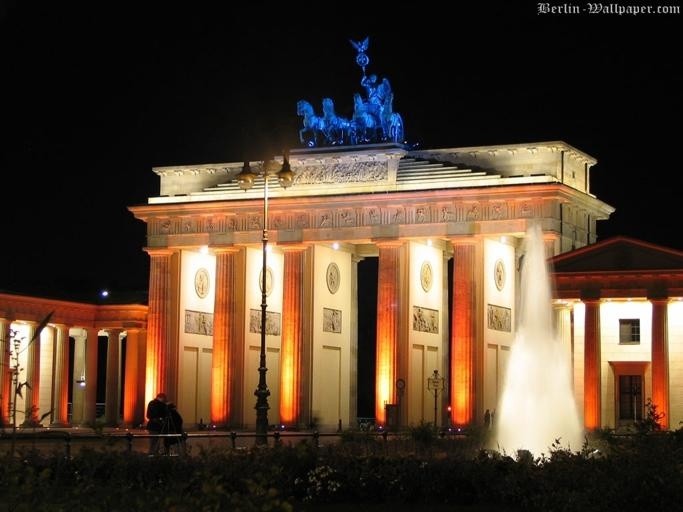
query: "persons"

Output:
[146,391,183,458]
[484,409,490,429]
[361,74,390,107]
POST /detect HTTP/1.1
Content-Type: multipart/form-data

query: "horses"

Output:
[297,92,397,147]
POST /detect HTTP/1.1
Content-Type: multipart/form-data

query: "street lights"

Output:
[232,137,298,463]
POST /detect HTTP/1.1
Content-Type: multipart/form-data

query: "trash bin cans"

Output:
[386,404,397,427]
